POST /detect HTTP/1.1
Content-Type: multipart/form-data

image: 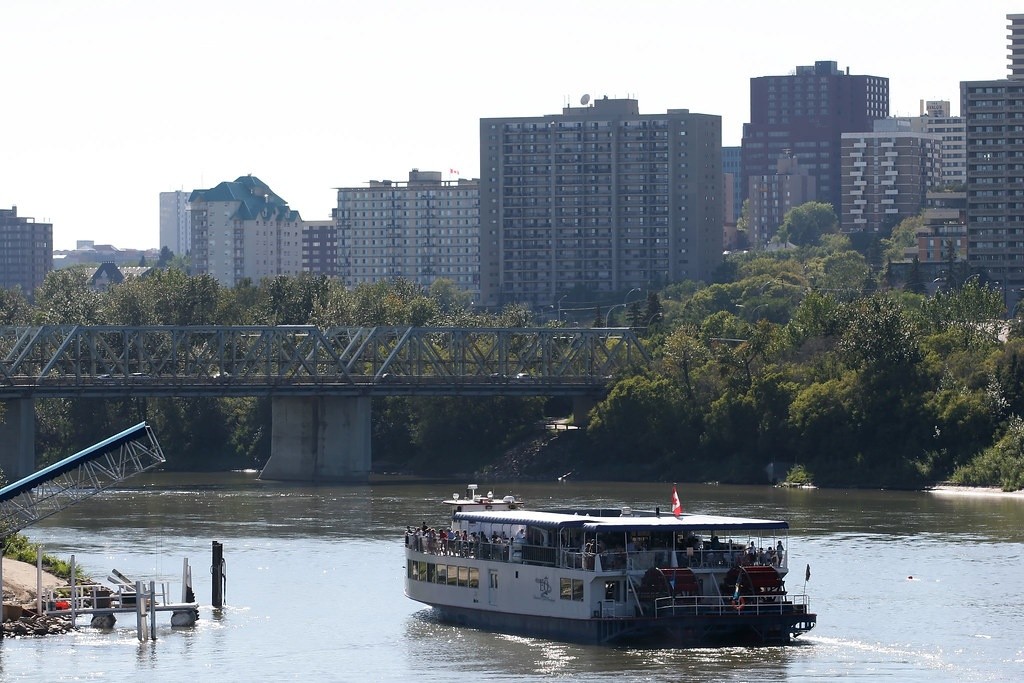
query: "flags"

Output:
[671,486,682,517]
[805,565,811,581]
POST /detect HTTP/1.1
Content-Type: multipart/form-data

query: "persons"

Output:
[404,522,525,562]
[559,531,784,567]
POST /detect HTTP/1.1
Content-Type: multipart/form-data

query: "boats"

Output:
[401,484,817,646]
